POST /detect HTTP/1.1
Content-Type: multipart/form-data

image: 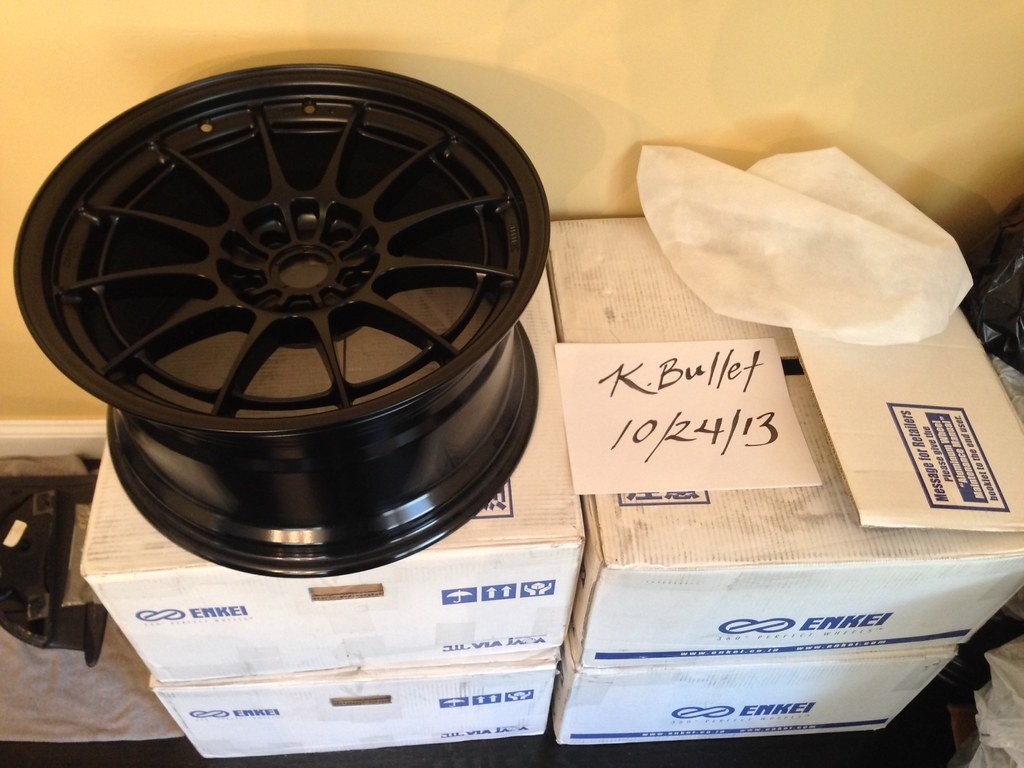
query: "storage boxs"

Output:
[545,216,1024,669]
[80,268,586,684]
[552,636,958,747]
[150,647,561,758]
